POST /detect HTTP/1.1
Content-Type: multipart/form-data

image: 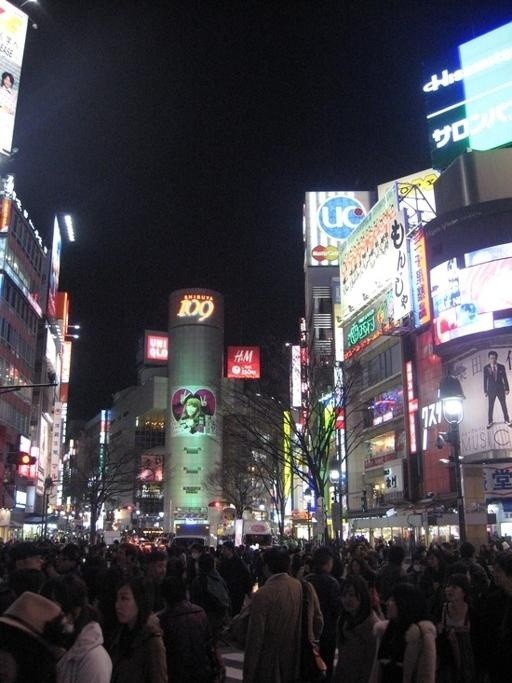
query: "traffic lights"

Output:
[8,452,34,465]
[209,501,230,508]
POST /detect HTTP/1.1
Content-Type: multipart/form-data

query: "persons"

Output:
[173,390,210,434]
[1,528,511,683]
[0,72,18,94]
[484,351,511,429]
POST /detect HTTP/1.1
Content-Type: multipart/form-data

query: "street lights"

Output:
[302,487,314,538]
[41,475,55,538]
[258,499,266,521]
[330,460,341,541]
[437,374,466,547]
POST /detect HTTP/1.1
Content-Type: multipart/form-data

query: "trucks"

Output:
[173,515,210,553]
[233,519,274,552]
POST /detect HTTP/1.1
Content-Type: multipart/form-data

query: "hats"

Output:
[0,592,73,660]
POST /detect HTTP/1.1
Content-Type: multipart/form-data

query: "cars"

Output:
[44,524,175,551]
[217,538,234,547]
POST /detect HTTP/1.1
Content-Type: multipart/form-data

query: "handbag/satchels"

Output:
[300,638,321,682]
